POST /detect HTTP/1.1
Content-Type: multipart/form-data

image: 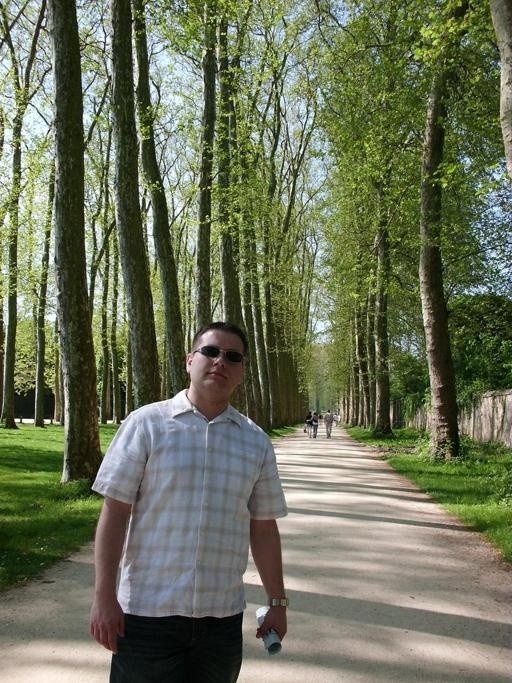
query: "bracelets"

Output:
[268,598,289,607]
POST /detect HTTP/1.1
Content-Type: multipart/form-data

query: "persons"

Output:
[306,412,319,438]
[323,409,334,438]
[333,413,338,427]
[88,320,289,683]
[305,411,312,438]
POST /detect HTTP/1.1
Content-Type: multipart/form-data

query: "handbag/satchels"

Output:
[304,428,307,433]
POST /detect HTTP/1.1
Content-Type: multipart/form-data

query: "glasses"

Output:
[193,346,244,364]
